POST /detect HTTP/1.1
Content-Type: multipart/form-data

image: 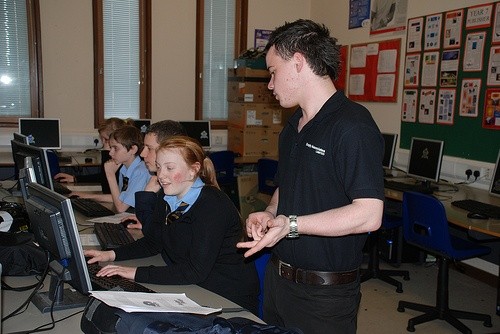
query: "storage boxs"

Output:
[227,67,280,103]
[236,56,267,70]
[227,126,284,163]
[244,192,272,220]
[227,101,301,130]
[236,172,259,219]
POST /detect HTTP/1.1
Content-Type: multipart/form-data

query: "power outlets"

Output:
[464,164,481,183]
[215,135,223,145]
[90,135,103,147]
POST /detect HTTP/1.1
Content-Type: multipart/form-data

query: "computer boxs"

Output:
[378,216,422,263]
[362,231,378,254]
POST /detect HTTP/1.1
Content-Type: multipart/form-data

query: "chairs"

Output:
[253,252,271,320]
[253,158,280,198]
[359,215,410,293]
[208,150,241,214]
[395,190,494,334]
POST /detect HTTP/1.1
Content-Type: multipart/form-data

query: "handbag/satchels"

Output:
[80,292,234,334]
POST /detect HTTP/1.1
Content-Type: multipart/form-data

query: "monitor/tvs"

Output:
[134,118,151,134]
[18,118,62,150]
[11,139,54,202]
[488,152,500,197]
[13,132,28,144]
[406,137,445,190]
[26,182,93,313]
[380,133,397,169]
[177,120,211,148]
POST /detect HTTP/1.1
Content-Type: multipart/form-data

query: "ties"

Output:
[165,201,190,224]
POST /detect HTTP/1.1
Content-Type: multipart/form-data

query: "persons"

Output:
[54,118,128,191]
[81,135,259,317]
[69,126,156,213]
[122,120,186,238]
[236,18,385,334]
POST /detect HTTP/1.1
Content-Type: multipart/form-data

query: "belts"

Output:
[272,256,361,287]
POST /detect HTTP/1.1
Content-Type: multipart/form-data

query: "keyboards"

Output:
[85,262,158,293]
[53,181,72,194]
[451,198,500,220]
[70,198,115,216]
[385,181,432,195]
[92,223,137,252]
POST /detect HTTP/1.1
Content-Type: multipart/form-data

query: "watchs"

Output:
[286,214,300,237]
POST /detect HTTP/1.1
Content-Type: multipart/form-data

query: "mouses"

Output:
[84,256,99,265]
[67,195,79,199]
[384,173,393,177]
[52,177,67,183]
[467,212,488,219]
[120,220,137,227]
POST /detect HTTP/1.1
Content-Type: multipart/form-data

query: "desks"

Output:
[74,152,102,177]
[0,152,82,180]
[0,180,267,334]
[381,167,500,319]
